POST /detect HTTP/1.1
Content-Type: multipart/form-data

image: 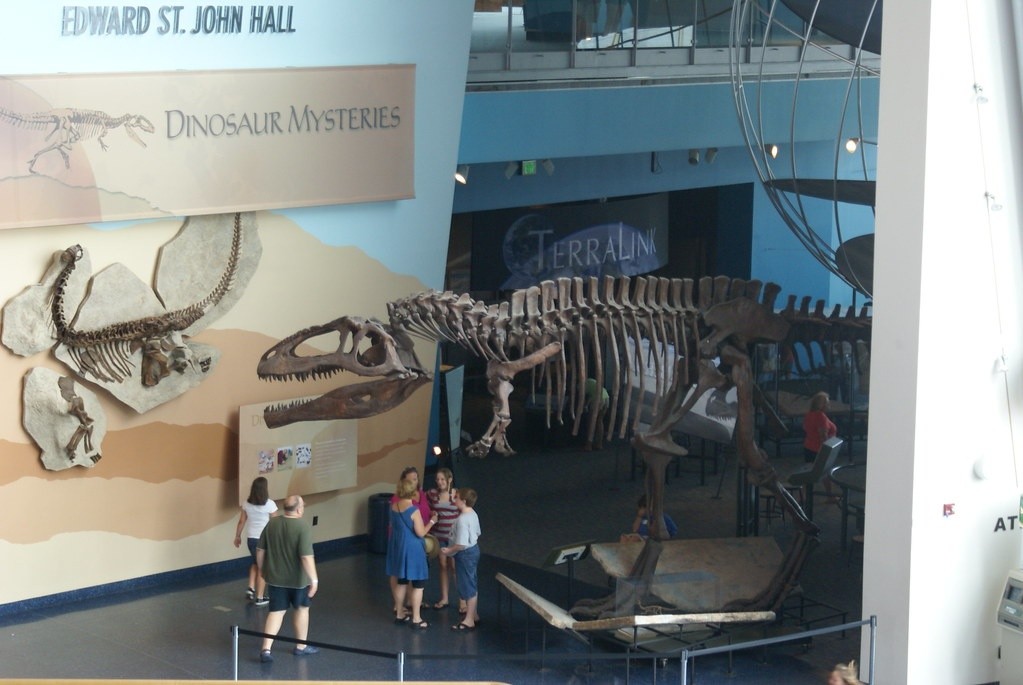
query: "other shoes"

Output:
[255,597,270,606]
[246,587,256,600]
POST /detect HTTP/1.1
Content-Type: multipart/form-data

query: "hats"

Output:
[424,534,440,559]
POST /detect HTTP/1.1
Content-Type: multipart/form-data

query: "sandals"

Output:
[411,620,431,629]
[395,614,412,625]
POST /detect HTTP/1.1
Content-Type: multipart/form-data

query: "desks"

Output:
[829,461,866,553]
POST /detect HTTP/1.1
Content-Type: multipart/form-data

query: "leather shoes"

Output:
[294,645,319,655]
[260,649,273,662]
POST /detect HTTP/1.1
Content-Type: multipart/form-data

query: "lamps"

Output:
[455,166,470,185]
[703,148,718,164]
[761,145,777,159]
[689,149,699,166]
[846,138,859,154]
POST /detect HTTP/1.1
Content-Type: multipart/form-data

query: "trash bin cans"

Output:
[368,492,393,554]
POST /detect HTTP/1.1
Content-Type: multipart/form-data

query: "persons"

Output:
[234,477,279,605]
[577,378,610,452]
[256,495,320,662]
[802,392,838,504]
[632,493,678,540]
[386,466,483,632]
[778,341,794,379]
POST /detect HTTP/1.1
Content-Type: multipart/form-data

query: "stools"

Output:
[758,481,803,525]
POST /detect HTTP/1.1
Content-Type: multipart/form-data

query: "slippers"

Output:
[433,601,449,609]
[461,615,480,625]
[393,606,412,615]
[592,445,602,450]
[406,600,431,609]
[451,622,475,632]
[578,447,592,452]
[459,606,467,613]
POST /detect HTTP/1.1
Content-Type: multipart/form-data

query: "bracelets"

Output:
[310,578,318,583]
[429,519,435,525]
